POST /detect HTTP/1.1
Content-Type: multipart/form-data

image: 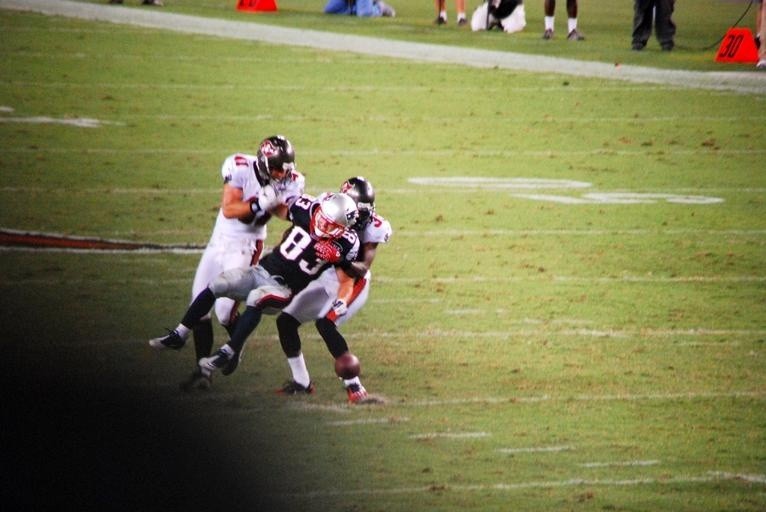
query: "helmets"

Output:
[340,177,374,230]
[256,135,295,180]
[314,192,358,238]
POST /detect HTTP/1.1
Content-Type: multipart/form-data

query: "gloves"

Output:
[258,185,282,210]
[314,241,344,264]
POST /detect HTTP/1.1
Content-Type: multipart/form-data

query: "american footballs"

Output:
[335,354,359,378]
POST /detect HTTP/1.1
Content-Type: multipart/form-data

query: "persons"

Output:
[107,0,165,6]
[179,134,306,391]
[432,0,468,26]
[147,190,363,373]
[631,0,676,52]
[273,175,393,405]
[470,0,527,33]
[755,0,766,68]
[542,0,585,41]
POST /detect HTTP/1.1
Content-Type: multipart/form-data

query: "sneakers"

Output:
[435,17,447,24]
[659,40,673,50]
[632,42,645,49]
[343,383,368,403]
[277,379,317,396]
[458,20,467,25]
[543,29,553,39]
[147,327,238,392]
[568,29,585,40]
